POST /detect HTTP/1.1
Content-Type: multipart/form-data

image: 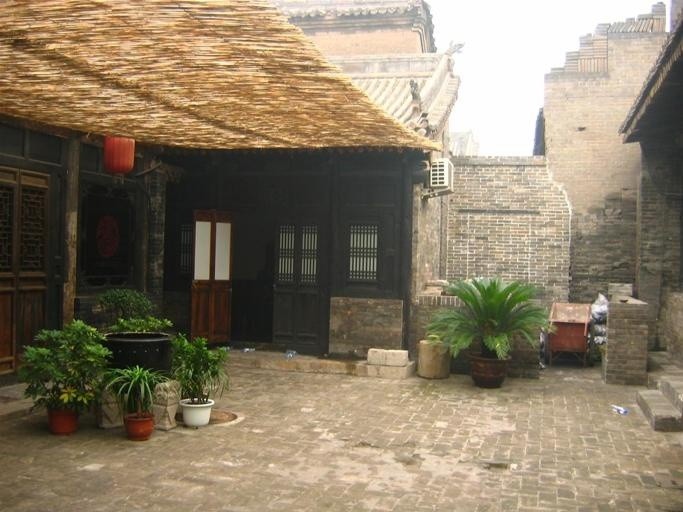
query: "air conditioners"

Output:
[429,158,454,191]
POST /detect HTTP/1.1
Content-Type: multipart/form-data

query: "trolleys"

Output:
[543,302,591,368]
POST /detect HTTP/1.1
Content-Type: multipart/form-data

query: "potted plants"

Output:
[18,288,230,442]
[423,276,559,389]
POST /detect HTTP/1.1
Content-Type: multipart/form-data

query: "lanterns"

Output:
[104,136,135,184]
